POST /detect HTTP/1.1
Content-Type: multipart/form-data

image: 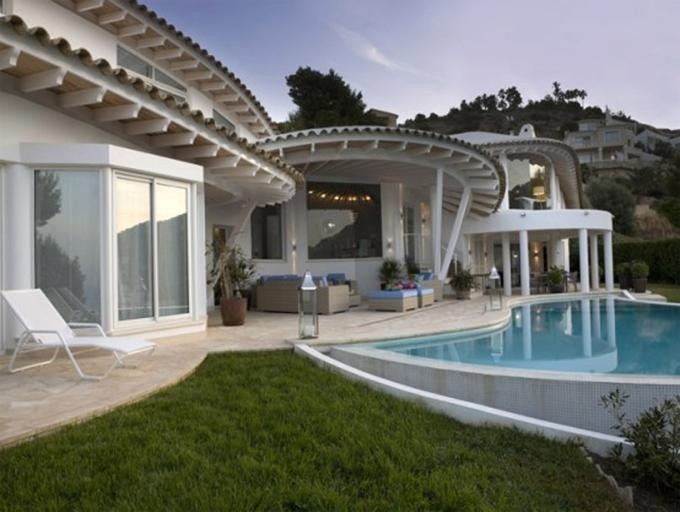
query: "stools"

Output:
[368,288,434,311]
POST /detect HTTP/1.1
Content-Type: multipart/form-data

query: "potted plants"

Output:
[378,258,401,291]
[547,265,564,293]
[615,259,650,293]
[449,269,474,300]
[204,232,256,325]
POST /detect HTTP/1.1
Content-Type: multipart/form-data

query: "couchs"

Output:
[255,273,360,313]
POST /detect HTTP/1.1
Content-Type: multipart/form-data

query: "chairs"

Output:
[1,288,157,381]
